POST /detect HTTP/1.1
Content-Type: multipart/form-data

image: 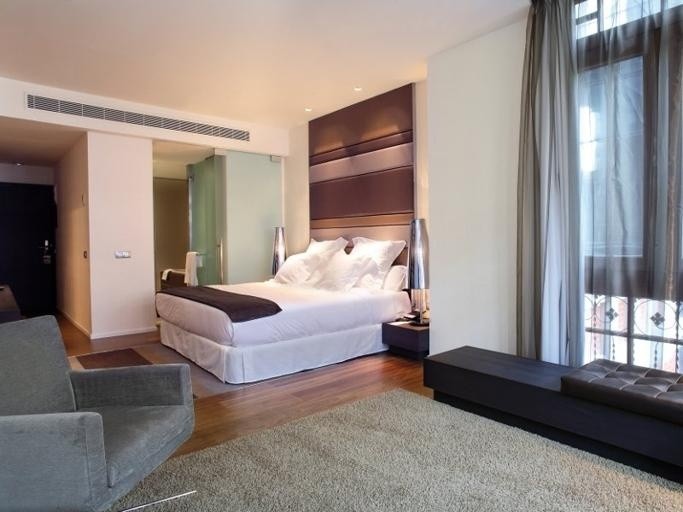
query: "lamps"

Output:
[409,218,429,326]
[272,226,286,276]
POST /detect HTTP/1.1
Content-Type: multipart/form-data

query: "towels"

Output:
[183,251,203,288]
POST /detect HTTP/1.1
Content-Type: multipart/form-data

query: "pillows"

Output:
[271,237,407,293]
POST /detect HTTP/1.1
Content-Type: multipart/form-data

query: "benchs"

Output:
[423,345,683,468]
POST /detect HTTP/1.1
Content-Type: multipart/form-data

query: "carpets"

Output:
[104,387,683,512]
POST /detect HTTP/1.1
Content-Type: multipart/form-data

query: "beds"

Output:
[155,246,411,385]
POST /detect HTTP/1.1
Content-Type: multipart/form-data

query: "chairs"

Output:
[0,315,198,512]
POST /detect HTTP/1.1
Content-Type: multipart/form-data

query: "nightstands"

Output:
[382,320,429,360]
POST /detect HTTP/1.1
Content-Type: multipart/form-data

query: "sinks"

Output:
[172,268,186,275]
